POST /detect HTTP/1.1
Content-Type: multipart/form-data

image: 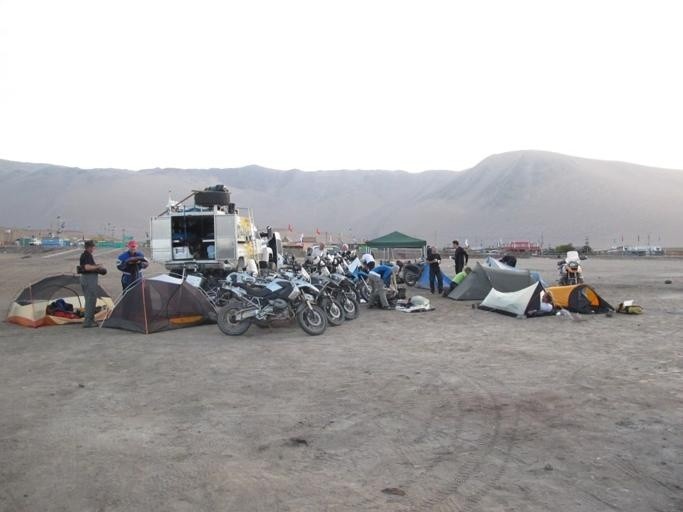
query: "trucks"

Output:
[150,205,284,278]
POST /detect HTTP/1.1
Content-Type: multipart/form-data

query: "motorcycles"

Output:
[214,243,425,336]
[557,251,584,286]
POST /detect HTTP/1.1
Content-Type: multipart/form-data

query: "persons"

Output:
[449,240,469,274]
[116,240,149,290]
[427,245,444,294]
[77,240,107,327]
[360,253,401,311]
[237,242,274,278]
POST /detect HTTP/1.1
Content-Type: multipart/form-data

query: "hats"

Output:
[84,240,95,247]
[127,239,138,250]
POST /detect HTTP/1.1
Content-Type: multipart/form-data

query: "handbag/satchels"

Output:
[616,302,643,313]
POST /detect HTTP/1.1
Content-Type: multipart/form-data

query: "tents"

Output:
[6,272,115,328]
[100,274,219,334]
[447,256,616,318]
